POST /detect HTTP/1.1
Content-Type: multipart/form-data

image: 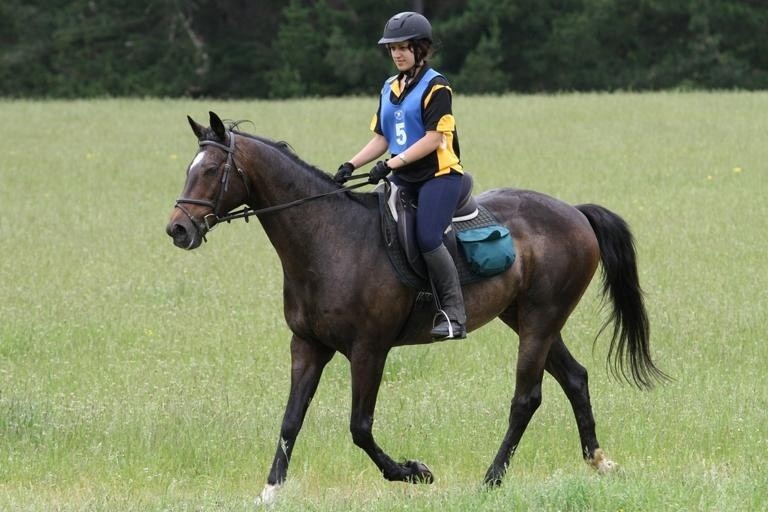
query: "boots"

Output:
[422,239,468,337]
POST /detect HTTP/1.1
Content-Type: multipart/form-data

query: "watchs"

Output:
[396,152,408,166]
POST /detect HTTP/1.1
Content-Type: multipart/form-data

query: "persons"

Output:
[333,6,466,340]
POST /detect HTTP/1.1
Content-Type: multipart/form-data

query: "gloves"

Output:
[368,159,392,184]
[335,162,355,184]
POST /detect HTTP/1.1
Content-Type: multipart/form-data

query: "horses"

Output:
[165,111,679,507]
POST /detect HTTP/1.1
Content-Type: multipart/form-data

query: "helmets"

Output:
[378,11,433,45]
